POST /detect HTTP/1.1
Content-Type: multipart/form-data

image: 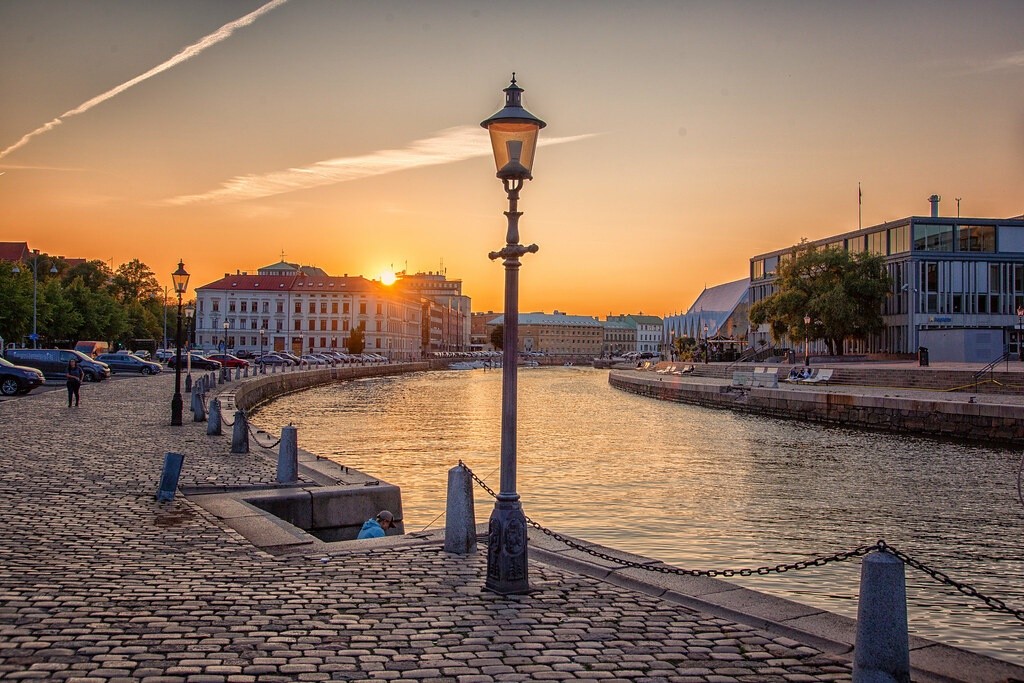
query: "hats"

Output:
[377,511,397,529]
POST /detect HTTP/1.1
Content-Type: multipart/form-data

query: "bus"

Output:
[73,340,109,361]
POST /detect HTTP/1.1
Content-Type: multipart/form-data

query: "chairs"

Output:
[784,369,814,385]
[766,368,778,373]
[801,369,833,386]
[754,367,765,373]
[635,362,651,372]
[656,366,677,375]
[673,365,696,377]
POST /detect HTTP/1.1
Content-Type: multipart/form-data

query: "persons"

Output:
[789,367,811,380]
[637,361,642,367]
[681,365,695,374]
[66,359,84,408]
[357,510,396,539]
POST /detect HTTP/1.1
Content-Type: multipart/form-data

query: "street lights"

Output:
[150,285,176,364]
[299,330,304,363]
[410,340,413,363]
[610,343,613,358]
[360,335,365,366]
[803,314,811,366]
[169,257,192,427]
[183,299,196,394]
[222,317,231,376]
[11,254,59,349]
[600,343,603,359]
[258,326,264,373]
[331,334,336,367]
[703,323,709,364]
[479,70,549,595]
[1016,305,1024,360]
[388,338,392,363]
[670,328,674,363]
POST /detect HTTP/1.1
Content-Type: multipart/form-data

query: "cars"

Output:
[154,346,184,363]
[134,349,152,361]
[620,350,661,360]
[227,348,387,367]
[95,352,164,377]
[205,352,250,369]
[116,350,133,355]
[167,353,221,371]
[426,350,547,359]
[0,355,45,397]
[4,346,111,383]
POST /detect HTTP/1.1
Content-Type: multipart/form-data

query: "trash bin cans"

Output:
[918,345,929,367]
[788,350,795,364]
